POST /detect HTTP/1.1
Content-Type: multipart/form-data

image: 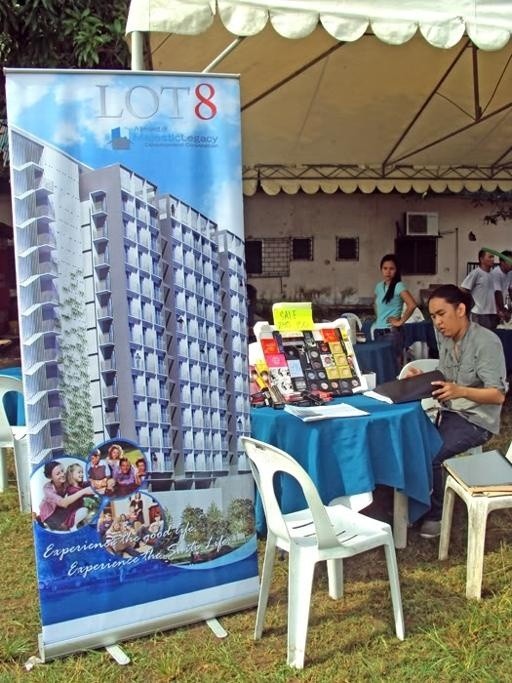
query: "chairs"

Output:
[0,372,36,519]
[239,436,411,671]
[436,432,512,605]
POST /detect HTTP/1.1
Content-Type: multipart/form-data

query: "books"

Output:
[443,448,512,498]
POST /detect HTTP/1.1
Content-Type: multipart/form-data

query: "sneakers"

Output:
[419,520,441,538]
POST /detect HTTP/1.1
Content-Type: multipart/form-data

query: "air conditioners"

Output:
[402,208,440,240]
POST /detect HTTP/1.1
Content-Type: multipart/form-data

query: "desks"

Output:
[238,313,433,552]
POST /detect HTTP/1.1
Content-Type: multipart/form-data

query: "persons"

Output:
[247,284,264,337]
[32,443,164,558]
[490,250,512,323]
[385,283,509,539]
[461,250,498,331]
[373,253,418,375]
